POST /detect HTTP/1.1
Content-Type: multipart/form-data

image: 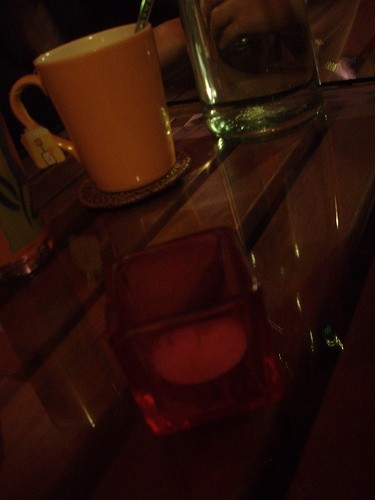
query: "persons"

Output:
[152,0,358,101]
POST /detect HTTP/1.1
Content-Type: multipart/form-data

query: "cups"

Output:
[9,18,176,194]
[104,225,285,440]
[1,111,53,278]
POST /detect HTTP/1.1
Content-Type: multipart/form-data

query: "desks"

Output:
[1,79,374,500]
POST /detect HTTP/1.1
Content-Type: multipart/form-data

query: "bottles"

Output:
[177,0,323,141]
